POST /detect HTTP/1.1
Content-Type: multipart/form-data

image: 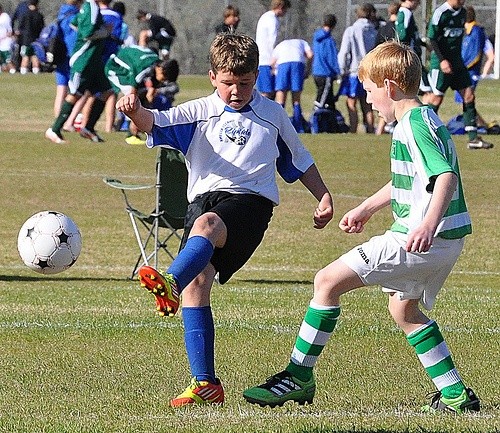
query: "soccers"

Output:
[16,210,83,275]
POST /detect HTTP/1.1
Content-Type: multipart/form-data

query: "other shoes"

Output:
[64,124,77,132]
[31,66,42,73]
[125,134,146,145]
[44,128,68,145]
[80,128,105,142]
[19,66,29,74]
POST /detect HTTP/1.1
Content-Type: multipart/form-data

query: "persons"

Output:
[0,0,179,145]
[256,0,292,100]
[215,4,240,33]
[244,28,480,411]
[115,32,333,407]
[269,0,500,148]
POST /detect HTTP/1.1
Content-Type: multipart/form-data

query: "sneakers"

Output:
[242,369,317,408]
[168,378,224,407]
[137,264,182,319]
[467,136,494,150]
[418,388,481,414]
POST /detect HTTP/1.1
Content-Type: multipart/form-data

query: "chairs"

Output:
[102,146,188,282]
